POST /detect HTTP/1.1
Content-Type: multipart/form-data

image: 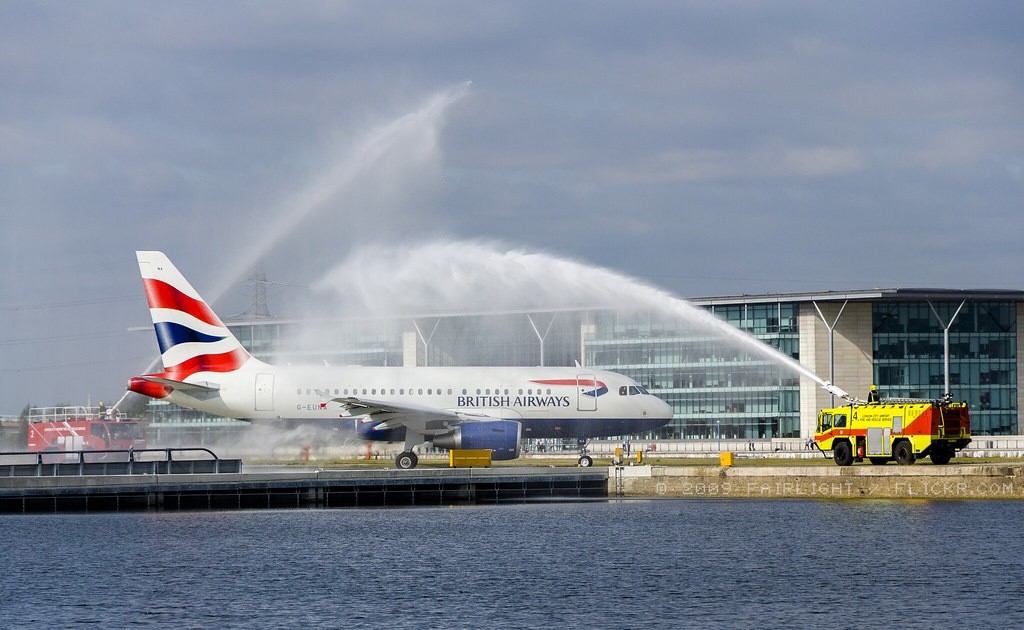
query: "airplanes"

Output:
[126,248,677,469]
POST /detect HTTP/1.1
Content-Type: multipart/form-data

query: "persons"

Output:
[99,402,106,420]
[867,385,881,405]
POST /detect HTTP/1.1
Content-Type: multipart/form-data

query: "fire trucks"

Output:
[813,394,973,466]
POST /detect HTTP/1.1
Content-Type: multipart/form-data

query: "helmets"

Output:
[870,385,877,391]
